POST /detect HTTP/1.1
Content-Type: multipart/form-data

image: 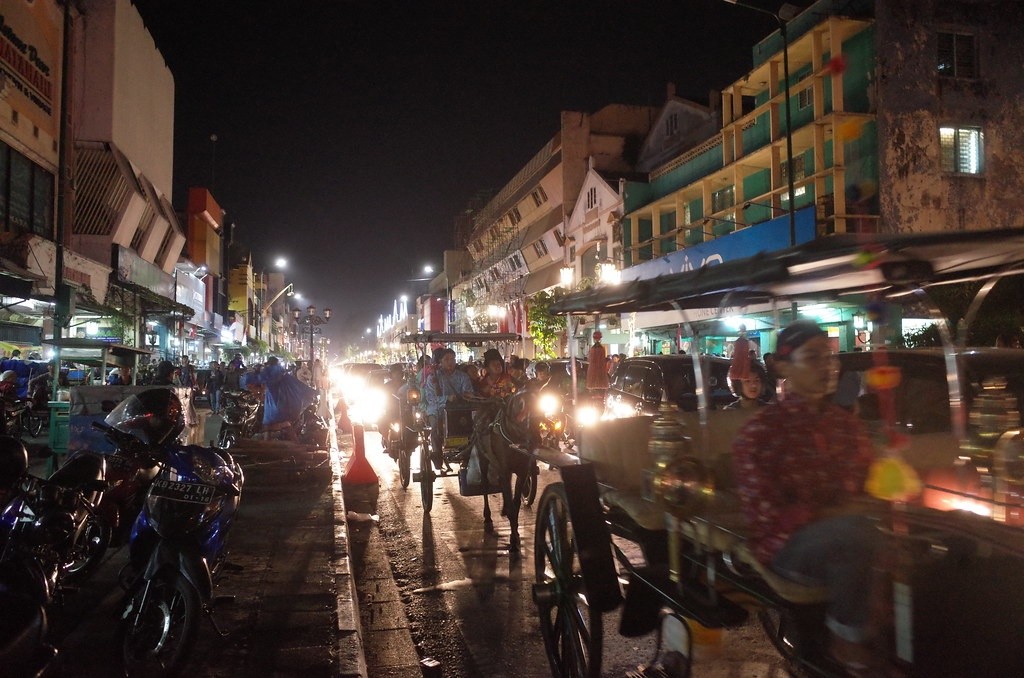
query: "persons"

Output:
[9,349,328,414]
[391,318,925,678]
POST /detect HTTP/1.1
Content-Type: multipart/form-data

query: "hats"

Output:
[2,370,15,382]
[483,348,503,368]
[774,317,829,361]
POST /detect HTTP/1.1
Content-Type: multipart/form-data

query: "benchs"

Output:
[577,411,740,536]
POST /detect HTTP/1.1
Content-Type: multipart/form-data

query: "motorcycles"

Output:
[103,387,246,678]
[1,434,117,678]
[379,407,402,461]
[217,385,260,453]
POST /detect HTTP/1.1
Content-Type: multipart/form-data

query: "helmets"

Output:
[105,388,185,451]
[389,363,405,379]
[535,362,549,376]
[726,358,767,398]
[566,361,581,376]
[143,442,243,541]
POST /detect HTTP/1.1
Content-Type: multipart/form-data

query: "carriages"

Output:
[400,331,547,550]
[527,220,1024,678]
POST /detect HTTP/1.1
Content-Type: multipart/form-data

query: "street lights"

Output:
[293,307,331,390]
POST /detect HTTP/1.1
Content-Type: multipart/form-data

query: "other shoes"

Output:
[431,452,443,470]
[823,648,875,678]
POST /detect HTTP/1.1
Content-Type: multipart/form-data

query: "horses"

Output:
[469,376,550,554]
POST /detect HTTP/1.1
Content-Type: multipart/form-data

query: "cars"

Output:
[338,363,391,424]
